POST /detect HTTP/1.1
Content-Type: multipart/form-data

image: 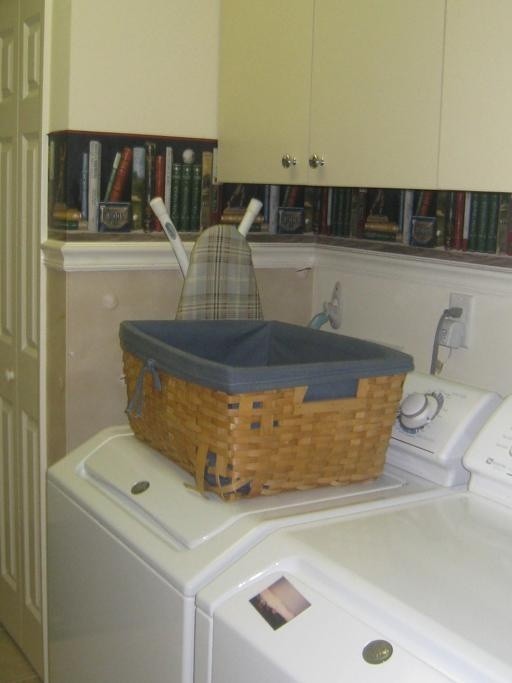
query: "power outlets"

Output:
[447,292,473,350]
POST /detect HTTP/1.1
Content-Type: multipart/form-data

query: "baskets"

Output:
[119,320,414,502]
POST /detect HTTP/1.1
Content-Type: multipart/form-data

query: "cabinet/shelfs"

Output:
[43,132,512,269]
[439,0,511,192]
[47,1,218,143]
[218,0,447,189]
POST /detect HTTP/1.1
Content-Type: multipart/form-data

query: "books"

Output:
[220,184,510,259]
[50,136,223,234]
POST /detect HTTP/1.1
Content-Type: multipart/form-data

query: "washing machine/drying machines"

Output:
[192,396,511,682]
[47,369,502,683]
